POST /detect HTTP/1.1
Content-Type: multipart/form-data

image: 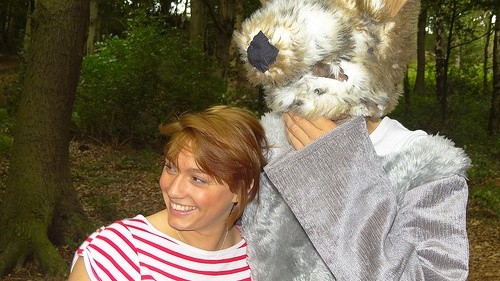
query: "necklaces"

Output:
[176,220,228,250]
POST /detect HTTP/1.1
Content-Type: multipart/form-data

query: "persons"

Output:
[69,106,278,281]
[232,1,470,280]
